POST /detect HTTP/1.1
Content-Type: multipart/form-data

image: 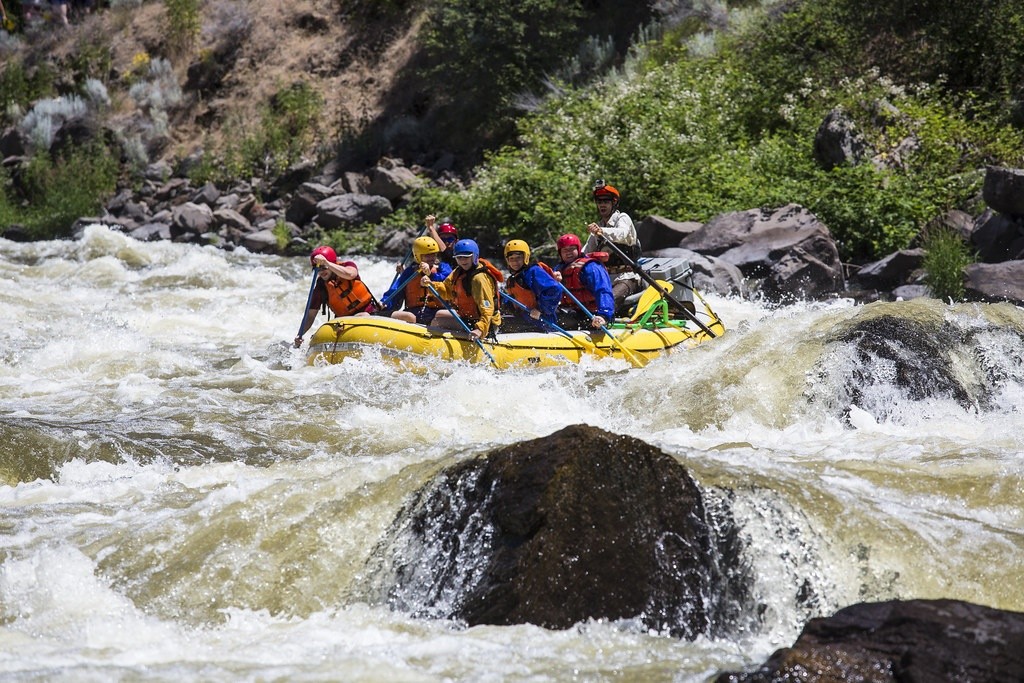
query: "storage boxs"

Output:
[637,257,695,303]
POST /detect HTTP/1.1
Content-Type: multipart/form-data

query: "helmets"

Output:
[412,236,440,265]
[557,233,582,258]
[452,238,480,266]
[593,185,620,198]
[504,239,531,266]
[437,222,458,238]
[311,246,337,266]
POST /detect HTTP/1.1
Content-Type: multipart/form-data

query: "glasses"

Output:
[441,236,456,243]
[595,198,613,204]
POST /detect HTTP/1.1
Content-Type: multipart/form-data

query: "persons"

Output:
[376,237,453,325]
[500,240,562,333]
[396,214,458,276]
[554,235,614,329]
[581,179,640,314]
[295,247,417,347]
[421,239,503,344]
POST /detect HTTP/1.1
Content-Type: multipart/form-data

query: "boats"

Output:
[305,258,727,376]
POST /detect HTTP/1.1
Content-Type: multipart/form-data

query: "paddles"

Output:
[296,262,320,348]
[388,221,427,291]
[426,282,500,368]
[629,279,674,323]
[382,266,423,305]
[498,290,608,359]
[592,230,717,337]
[557,281,650,370]
